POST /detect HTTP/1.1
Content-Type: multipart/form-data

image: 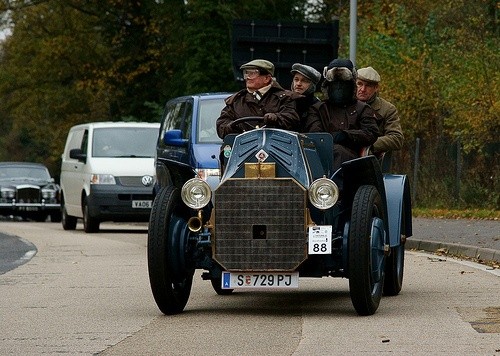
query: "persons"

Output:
[216,59,300,141]
[289,63,322,132]
[305,59,383,174]
[356,66,405,157]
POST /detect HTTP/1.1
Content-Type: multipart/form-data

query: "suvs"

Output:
[147,116,412,315]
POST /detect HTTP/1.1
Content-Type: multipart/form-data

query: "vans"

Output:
[60,121,161,233]
[154,92,238,204]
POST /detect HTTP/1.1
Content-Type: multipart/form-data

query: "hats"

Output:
[356,66,380,85]
[323,59,358,101]
[240,59,275,75]
[290,63,321,85]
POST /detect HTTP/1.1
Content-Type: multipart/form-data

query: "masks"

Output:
[332,86,349,100]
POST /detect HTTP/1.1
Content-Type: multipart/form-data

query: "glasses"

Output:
[242,69,260,80]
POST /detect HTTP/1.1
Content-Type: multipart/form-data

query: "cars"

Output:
[0,162,62,222]
